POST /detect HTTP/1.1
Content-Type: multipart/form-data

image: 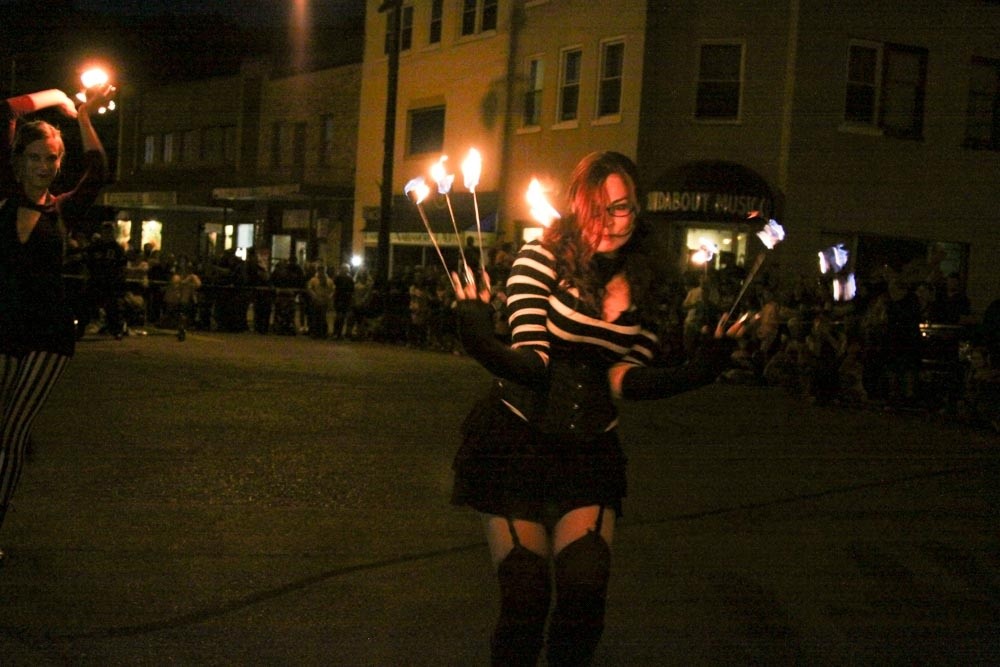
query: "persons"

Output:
[1,87,138,527]
[445,150,762,667]
[58,220,1000,433]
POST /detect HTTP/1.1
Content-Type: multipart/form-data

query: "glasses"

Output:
[590,204,636,217]
